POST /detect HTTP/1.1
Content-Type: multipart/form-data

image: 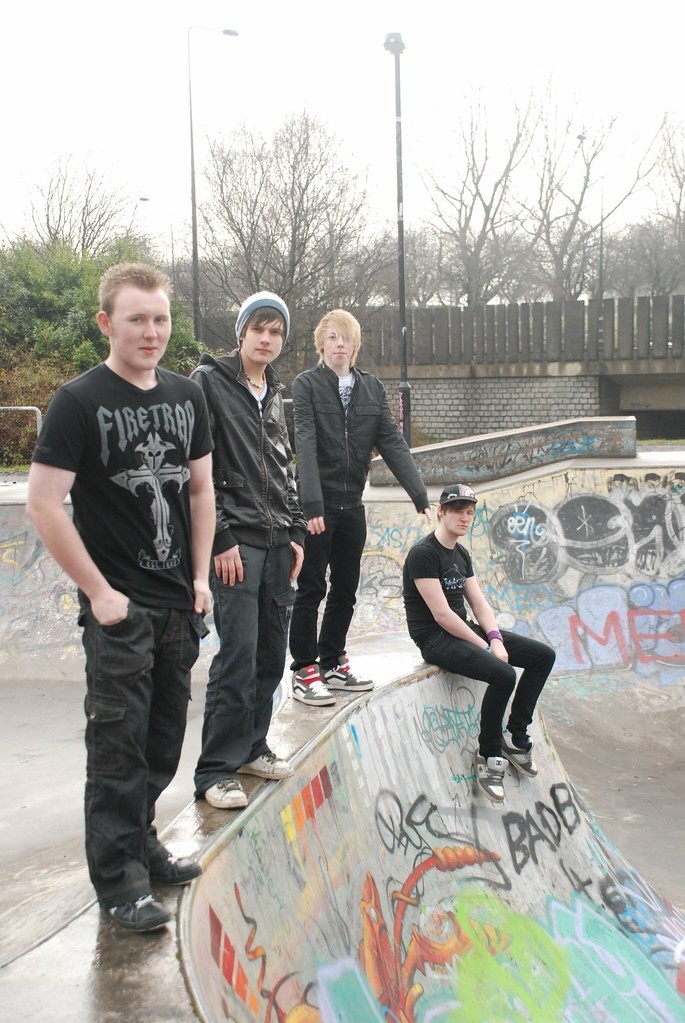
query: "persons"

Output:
[183,289,299,813]
[288,307,432,704]
[28,260,200,936]
[406,480,554,799]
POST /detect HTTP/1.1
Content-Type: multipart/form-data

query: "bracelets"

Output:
[487,630,504,642]
[486,646,492,654]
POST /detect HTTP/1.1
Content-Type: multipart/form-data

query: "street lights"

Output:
[187,25,239,342]
[382,32,411,449]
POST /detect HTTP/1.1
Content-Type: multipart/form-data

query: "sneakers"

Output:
[500,728,538,777]
[474,746,510,803]
[99,891,173,933]
[292,663,336,706]
[203,778,249,809]
[236,752,295,780]
[149,848,204,885]
[320,655,374,692]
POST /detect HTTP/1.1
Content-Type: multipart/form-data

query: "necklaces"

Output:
[241,367,267,390]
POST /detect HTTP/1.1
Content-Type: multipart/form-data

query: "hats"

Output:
[235,290,290,348]
[439,483,478,505]
